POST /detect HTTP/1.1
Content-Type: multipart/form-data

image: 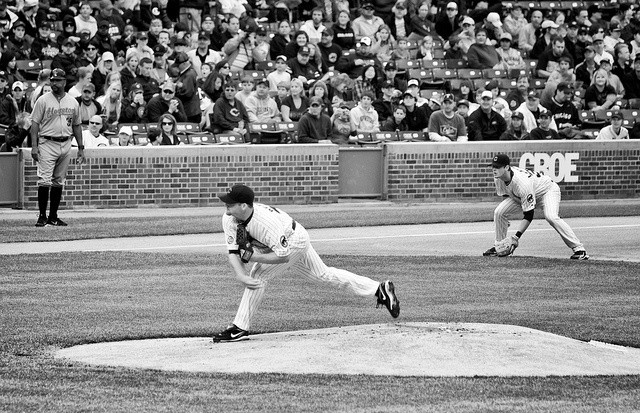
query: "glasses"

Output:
[65,22,73,26]
[99,26,107,29]
[162,121,172,125]
[362,6,373,10]
[90,120,100,125]
[38,22,50,28]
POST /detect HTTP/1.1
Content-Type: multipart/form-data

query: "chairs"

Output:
[134,135,159,143]
[119,122,148,132]
[376,131,396,139]
[409,68,430,80]
[578,109,595,120]
[355,132,370,139]
[239,71,264,79]
[398,131,425,139]
[451,79,472,90]
[558,2,585,10]
[188,133,212,142]
[396,58,420,68]
[176,123,199,131]
[485,67,507,79]
[172,134,187,144]
[228,71,243,81]
[38,59,52,67]
[422,88,445,97]
[456,68,483,79]
[252,123,274,132]
[538,1,560,9]
[215,133,243,144]
[434,40,443,48]
[147,121,165,130]
[425,60,444,68]
[531,79,546,87]
[260,22,303,36]
[519,1,541,9]
[455,58,471,67]
[433,64,456,79]
[407,41,416,49]
[255,60,276,69]
[473,81,491,88]
[598,110,617,119]
[623,109,635,123]
[15,60,41,68]
[500,79,519,87]
[524,60,538,68]
[278,121,302,132]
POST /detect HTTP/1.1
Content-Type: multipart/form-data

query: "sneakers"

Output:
[571,251,589,261]
[375,280,400,318]
[483,247,497,257]
[213,327,249,342]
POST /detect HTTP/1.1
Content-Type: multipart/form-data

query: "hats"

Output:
[540,111,552,117]
[203,15,213,20]
[527,90,540,97]
[101,52,114,61]
[567,19,578,28]
[457,99,469,107]
[98,1,116,10]
[600,58,611,64]
[14,22,25,27]
[98,21,109,26]
[360,37,372,45]
[24,6,36,11]
[134,30,148,41]
[403,89,417,98]
[592,33,603,42]
[462,17,474,25]
[256,78,269,87]
[62,39,75,47]
[82,83,95,92]
[339,103,351,110]
[541,21,559,28]
[153,45,166,55]
[0,71,7,80]
[486,12,503,27]
[511,112,523,119]
[590,5,603,11]
[130,83,143,92]
[500,32,512,41]
[557,82,571,93]
[489,154,511,167]
[610,21,622,31]
[447,2,458,9]
[612,111,624,120]
[162,81,175,92]
[199,30,210,38]
[584,46,596,52]
[407,78,419,86]
[381,80,394,87]
[24,0,39,6]
[172,52,188,68]
[309,96,322,107]
[219,186,254,204]
[320,28,336,37]
[299,46,311,56]
[443,94,456,101]
[479,90,494,99]
[12,81,24,90]
[275,55,287,61]
[397,2,406,8]
[431,93,443,106]
[384,62,398,71]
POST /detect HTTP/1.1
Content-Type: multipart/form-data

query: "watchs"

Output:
[30,67,85,227]
[78,144,84,148]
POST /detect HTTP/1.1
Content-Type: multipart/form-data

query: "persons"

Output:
[0,0,640,151]
[484,154,590,259]
[213,185,401,342]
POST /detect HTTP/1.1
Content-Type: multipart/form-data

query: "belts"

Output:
[42,135,70,142]
[292,221,296,229]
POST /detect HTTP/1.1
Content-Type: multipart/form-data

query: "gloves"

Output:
[236,224,253,263]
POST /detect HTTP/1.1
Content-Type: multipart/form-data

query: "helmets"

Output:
[50,68,66,80]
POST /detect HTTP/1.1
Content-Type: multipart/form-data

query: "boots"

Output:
[49,186,68,226]
[35,186,48,226]
